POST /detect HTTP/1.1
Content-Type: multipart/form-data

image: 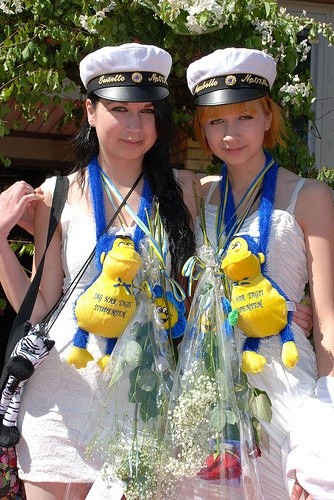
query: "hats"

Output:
[185,47,278,107]
[79,41,173,104]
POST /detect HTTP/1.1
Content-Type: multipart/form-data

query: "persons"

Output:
[17,42,334,499]
[0,41,314,500]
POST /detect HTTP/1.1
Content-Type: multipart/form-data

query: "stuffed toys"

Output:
[66,233,153,371]
[150,283,187,339]
[220,232,299,374]
[188,296,233,358]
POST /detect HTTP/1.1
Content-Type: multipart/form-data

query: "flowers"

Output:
[83,182,273,500]
